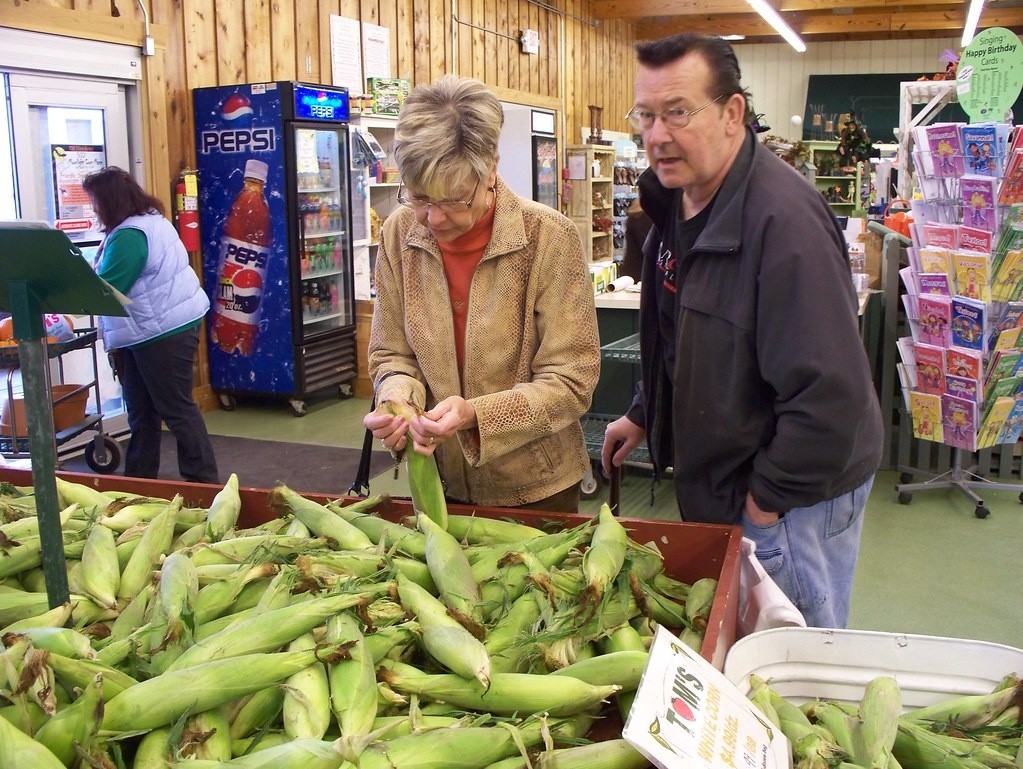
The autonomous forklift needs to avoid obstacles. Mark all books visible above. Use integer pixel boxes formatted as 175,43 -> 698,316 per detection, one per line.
896,122 -> 1023,452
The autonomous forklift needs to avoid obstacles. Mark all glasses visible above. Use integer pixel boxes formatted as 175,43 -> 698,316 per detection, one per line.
397,176 -> 480,211
624,93 -> 727,131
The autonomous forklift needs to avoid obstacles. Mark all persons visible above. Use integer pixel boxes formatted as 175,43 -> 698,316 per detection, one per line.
83,167 -> 220,484
621,198 -> 654,283
603,32 -> 883,629
367,75 -> 602,516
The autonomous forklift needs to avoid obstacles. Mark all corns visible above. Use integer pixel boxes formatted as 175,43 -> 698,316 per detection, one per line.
0,396 -> 718,769
744,672 -> 1023,769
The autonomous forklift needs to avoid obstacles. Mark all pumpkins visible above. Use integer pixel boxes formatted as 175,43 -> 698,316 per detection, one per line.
0,313 -> 74,355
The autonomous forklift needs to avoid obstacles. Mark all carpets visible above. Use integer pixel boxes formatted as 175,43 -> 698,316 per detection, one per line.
53,430 -> 397,494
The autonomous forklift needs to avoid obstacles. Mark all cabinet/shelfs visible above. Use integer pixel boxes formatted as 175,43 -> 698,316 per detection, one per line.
567,144 -> 616,263
892,148 -> 1022,518
348,114 -> 406,299
798,141 -> 861,217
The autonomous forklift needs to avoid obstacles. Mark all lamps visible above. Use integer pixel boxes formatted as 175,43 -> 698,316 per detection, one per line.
520,29 -> 538,54
959,0 -> 986,47
745,0 -> 807,52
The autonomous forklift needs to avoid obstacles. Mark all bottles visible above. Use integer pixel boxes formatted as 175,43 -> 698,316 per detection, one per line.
210,159 -> 271,357
297,154 -> 340,318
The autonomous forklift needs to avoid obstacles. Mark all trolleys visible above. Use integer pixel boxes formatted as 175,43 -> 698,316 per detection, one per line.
0,311 -> 124,475
577,329 -> 673,500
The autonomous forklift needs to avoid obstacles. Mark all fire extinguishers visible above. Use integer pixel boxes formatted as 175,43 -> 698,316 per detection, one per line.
173,165 -> 204,252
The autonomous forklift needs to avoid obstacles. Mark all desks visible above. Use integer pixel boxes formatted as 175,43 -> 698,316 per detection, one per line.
0,466 -> 743,769
594,288 -> 872,480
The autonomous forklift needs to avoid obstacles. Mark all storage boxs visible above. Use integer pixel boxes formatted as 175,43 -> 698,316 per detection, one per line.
367,78 -> 411,115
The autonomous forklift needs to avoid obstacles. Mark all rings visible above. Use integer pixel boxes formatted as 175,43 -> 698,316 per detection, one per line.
427,436 -> 433,446
381,439 -> 392,449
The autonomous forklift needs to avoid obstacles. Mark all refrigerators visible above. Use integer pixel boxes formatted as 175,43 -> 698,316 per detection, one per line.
497,103 -> 559,217
194,81 -> 359,393
